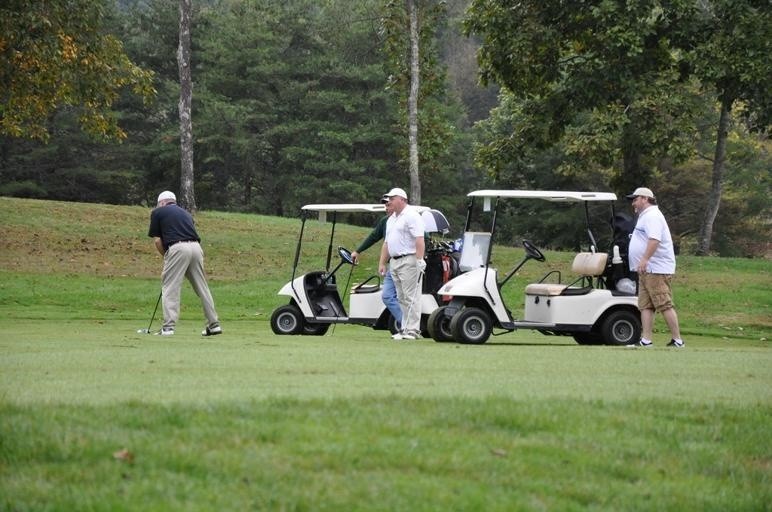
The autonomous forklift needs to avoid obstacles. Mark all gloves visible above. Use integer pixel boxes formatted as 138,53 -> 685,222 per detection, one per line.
417,259 -> 427,274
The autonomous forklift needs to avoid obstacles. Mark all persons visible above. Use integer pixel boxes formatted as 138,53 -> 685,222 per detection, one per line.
147,189 -> 223,337
625,186 -> 686,346
378,187 -> 428,340
350,198 -> 431,324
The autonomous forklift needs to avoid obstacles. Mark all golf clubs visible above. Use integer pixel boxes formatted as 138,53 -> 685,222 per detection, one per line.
144,292 -> 161,333
399,270 -> 423,335
428,227 -> 453,253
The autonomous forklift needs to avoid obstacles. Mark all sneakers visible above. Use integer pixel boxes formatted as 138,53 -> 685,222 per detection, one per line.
155,329 -> 176,336
202,324 -> 222,336
391,330 -> 420,340
626,338 -> 653,347
666,338 -> 685,348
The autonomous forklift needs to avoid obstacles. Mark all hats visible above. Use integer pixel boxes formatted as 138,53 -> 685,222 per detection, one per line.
157,190 -> 177,203
625,187 -> 654,199
380,187 -> 408,203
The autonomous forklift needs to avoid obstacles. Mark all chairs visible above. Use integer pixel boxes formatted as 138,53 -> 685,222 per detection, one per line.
351,275 -> 383,293
524,252 -> 609,297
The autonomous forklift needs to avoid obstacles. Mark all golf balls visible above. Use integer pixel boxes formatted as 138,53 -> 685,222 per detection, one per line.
136,329 -> 141,335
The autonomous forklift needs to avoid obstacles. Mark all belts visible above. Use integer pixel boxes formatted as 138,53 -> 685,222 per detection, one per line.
166,239 -> 183,250
393,254 -> 404,259
